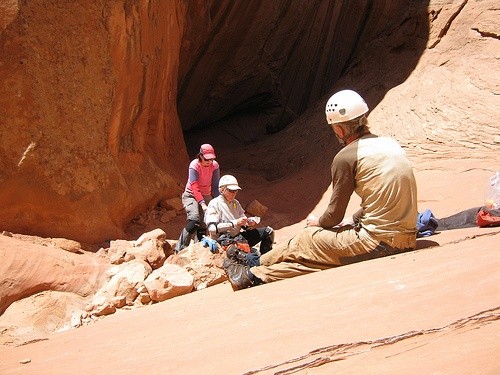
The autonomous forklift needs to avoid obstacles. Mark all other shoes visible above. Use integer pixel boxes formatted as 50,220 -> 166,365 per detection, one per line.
175,250 -> 178,255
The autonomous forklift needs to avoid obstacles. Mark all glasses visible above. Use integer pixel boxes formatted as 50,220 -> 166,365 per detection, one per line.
201,155 -> 214,161
221,187 -> 238,193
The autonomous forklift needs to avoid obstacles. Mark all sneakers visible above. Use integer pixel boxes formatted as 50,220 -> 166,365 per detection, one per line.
226,245 -> 249,266
222,258 -> 254,291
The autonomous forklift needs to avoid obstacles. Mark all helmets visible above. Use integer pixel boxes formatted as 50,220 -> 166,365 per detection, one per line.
325,89 -> 369,125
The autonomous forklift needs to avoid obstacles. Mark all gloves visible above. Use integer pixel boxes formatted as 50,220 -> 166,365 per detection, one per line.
202,237 -> 218,254
265,226 -> 274,234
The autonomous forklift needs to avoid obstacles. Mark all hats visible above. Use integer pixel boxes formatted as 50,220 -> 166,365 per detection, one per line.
200,144 -> 216,159
219,174 -> 242,191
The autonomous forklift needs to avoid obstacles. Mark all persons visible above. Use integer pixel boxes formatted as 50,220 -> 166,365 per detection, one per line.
174,144 -> 220,254
203,175 -> 276,256
223,90 -> 418,292
